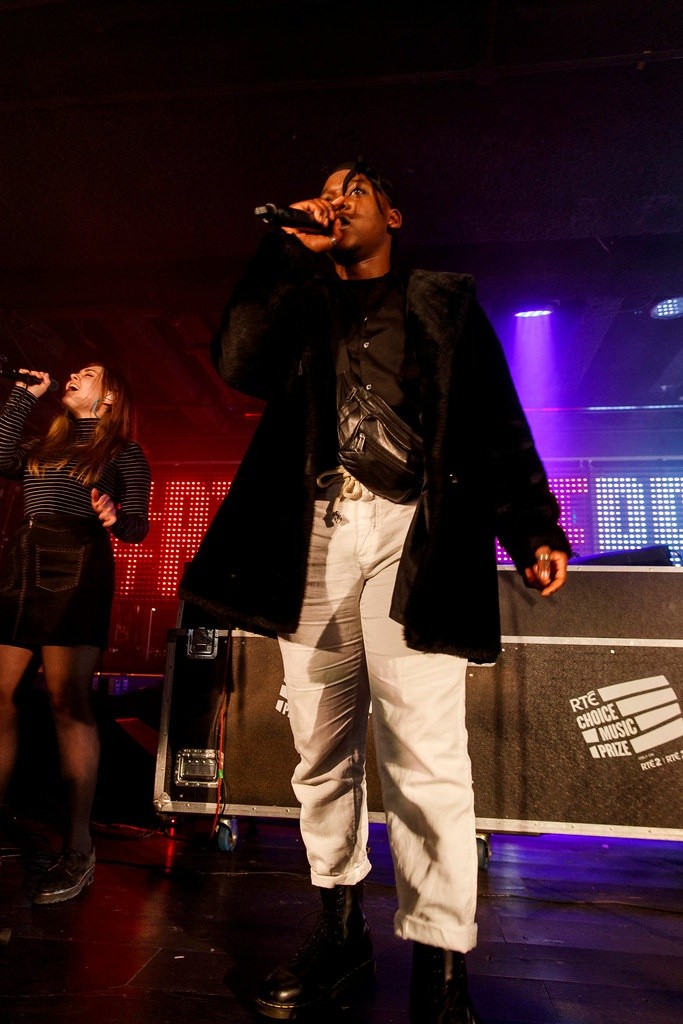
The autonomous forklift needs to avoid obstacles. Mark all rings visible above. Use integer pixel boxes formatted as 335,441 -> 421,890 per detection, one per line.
538,554 -> 550,560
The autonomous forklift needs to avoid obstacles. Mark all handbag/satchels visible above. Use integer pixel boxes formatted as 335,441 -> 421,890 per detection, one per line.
337,386 -> 427,505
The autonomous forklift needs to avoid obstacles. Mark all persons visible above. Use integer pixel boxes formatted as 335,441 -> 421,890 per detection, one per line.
177,162 -> 569,1023
0,360 -> 149,902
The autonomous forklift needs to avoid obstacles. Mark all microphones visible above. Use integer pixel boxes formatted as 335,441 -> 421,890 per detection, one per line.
0,367 -> 59,390
255,201 -> 335,236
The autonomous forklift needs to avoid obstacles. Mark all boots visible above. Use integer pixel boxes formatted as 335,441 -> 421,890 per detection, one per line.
409,943 -> 475,1024
255,880 -> 376,1020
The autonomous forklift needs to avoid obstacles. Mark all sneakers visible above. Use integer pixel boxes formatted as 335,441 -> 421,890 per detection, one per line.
26,842 -> 96,906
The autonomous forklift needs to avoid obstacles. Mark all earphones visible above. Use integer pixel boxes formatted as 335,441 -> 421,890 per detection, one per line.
106,395 -> 114,400
